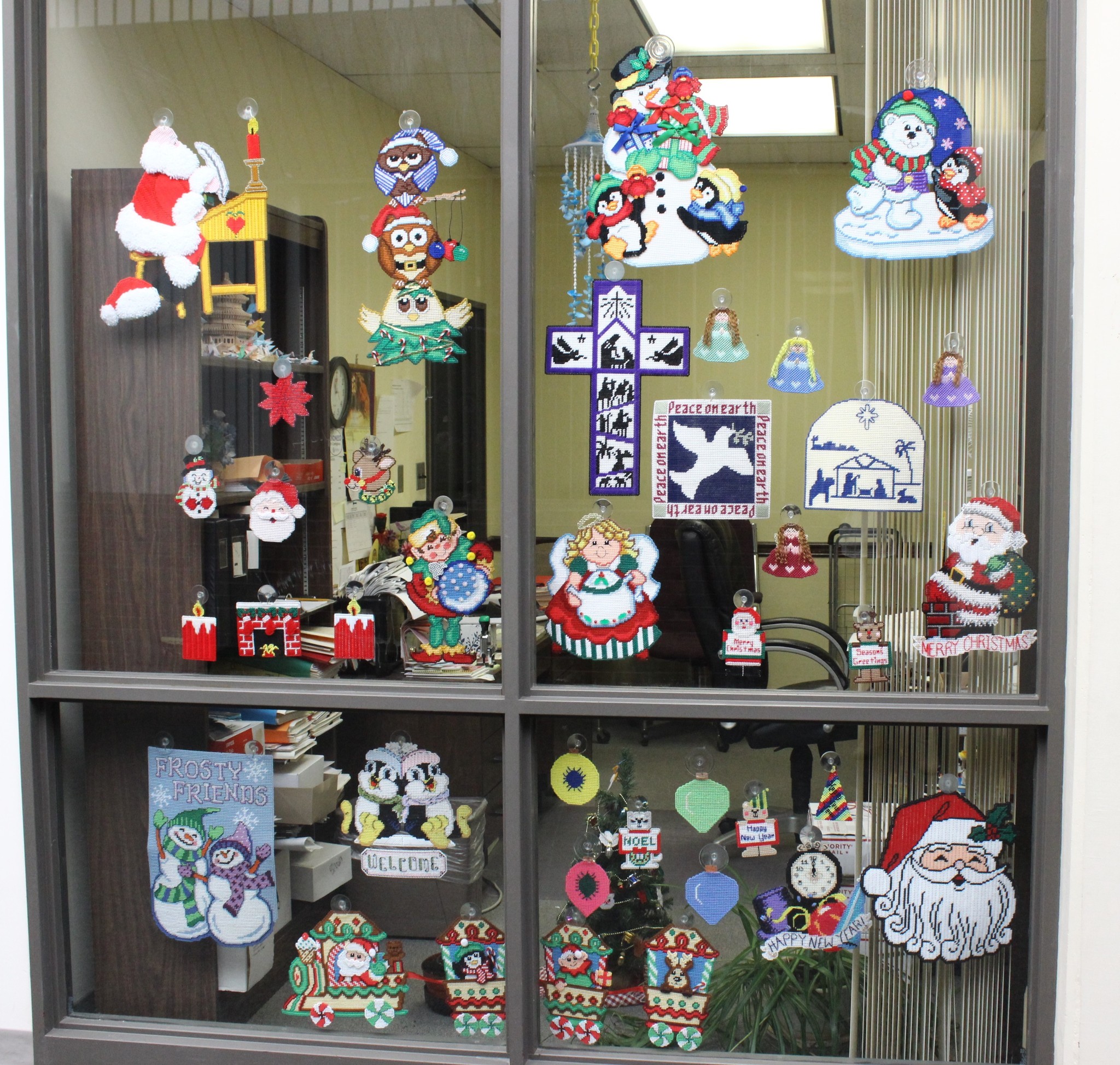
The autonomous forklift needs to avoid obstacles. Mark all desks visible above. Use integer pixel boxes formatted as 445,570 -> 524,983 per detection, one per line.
312,620 -> 592,880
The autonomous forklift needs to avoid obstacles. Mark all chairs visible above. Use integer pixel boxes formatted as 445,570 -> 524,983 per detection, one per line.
390,507 -> 430,524
412,501 -> 434,509
645,520 -> 760,754
674,520 -> 856,847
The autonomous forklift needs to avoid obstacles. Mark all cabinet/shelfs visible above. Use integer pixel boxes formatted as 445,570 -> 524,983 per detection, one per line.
71,166 -> 335,1028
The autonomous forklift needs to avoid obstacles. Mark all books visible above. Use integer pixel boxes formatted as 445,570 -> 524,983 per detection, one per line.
403,664 -> 490,679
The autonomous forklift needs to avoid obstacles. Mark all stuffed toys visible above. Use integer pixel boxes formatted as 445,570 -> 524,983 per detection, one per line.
92,129 -> 232,332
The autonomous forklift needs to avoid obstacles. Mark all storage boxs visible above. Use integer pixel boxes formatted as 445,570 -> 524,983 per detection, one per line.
807,802 -> 900,878
208,719 -> 265,755
274,849 -> 292,934
274,773 -> 338,825
337,592 -> 409,678
217,932 -> 273,993
290,841 -> 352,902
243,597 -> 337,656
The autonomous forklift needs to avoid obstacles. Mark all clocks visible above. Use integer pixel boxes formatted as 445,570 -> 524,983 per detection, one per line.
329,356 -> 352,428
785,841 -> 843,906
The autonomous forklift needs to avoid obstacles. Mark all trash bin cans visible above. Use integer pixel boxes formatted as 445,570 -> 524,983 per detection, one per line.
337,798 -> 488,937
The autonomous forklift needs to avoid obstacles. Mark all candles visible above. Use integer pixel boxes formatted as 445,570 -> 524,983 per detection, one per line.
246,128 -> 261,160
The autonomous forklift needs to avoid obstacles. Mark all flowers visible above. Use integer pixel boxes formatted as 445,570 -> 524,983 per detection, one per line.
374,512 -> 410,542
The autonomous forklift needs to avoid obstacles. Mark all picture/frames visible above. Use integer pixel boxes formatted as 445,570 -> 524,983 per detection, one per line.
342,354 -> 376,501
